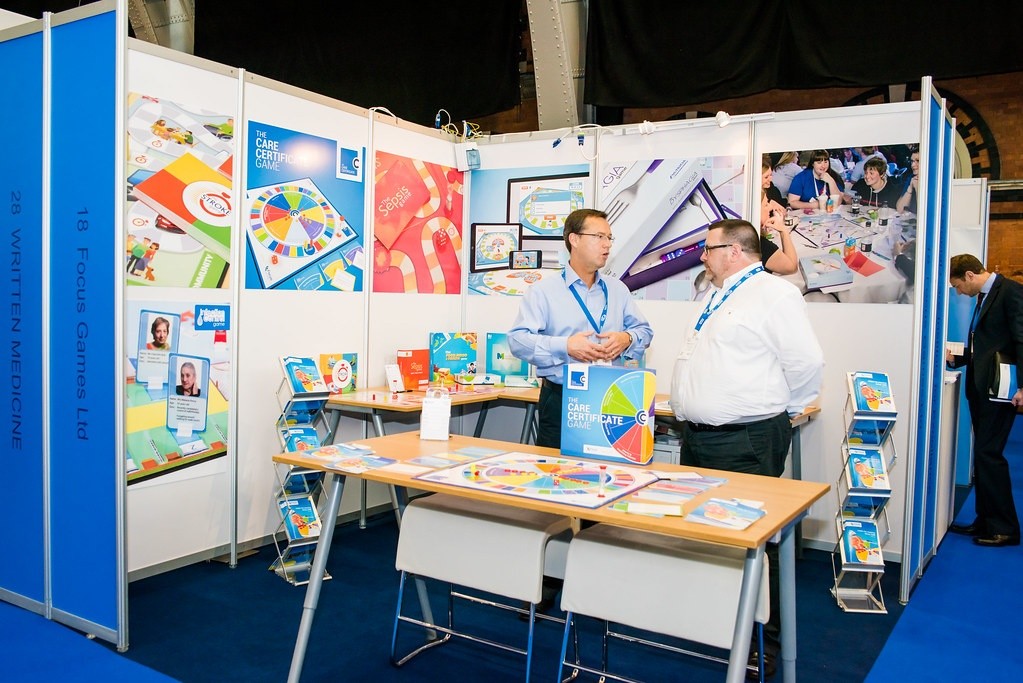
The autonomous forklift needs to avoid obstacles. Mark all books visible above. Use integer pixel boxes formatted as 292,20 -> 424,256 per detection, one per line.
841,518 -> 884,565
610,473 -> 769,532
278,496 -> 321,540
845,447 -> 891,489
849,370 -> 895,413
282,427 -> 322,469
283,355 -> 327,393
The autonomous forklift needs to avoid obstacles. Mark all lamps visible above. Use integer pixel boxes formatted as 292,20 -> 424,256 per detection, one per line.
639,121 -> 654,136
715,111 -> 732,127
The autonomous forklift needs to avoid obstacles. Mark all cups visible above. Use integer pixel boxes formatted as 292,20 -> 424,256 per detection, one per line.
817,196 -> 828,211
830,194 -> 840,211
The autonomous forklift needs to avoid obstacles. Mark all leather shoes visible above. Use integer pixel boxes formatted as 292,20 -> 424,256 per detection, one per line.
747,652 -> 776,679
973,532 -> 1020,546
949,522 -> 983,535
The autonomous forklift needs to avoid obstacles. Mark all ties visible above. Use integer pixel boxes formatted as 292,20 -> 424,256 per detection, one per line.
976,293 -> 986,315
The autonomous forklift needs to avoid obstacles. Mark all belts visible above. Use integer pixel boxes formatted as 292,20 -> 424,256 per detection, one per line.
543,377 -> 562,393
691,423 -> 745,430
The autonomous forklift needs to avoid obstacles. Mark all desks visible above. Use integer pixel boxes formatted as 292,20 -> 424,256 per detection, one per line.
498,388 -> 822,559
273,430 -> 831,683
768,205 -> 917,303
325,386 -> 505,528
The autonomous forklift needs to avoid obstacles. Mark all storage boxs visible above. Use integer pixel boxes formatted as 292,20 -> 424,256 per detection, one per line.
799,253 -> 853,289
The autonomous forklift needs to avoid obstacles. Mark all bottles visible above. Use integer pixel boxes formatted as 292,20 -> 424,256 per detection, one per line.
851,192 -> 861,216
861,222 -> 873,256
877,201 -> 890,238
783,203 -> 794,228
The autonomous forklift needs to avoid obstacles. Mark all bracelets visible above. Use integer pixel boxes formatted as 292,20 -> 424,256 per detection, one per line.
629,336 -> 632,342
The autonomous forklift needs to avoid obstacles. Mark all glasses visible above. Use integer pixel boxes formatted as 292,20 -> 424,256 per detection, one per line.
578,232 -> 616,244
908,159 -> 918,165
704,245 -> 744,256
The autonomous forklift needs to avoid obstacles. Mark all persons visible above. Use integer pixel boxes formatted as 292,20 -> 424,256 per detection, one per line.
671,218 -> 825,683
946,253 -> 1023,547
760,143 -> 919,305
175,361 -> 200,397
147,316 -> 170,351
508,209 -> 654,622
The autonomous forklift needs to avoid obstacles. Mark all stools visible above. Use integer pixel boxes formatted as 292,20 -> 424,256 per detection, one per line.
391,492 -> 582,683
557,523 -> 770,683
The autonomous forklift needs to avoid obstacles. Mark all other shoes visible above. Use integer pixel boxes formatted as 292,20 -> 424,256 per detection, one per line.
520,597 -> 551,623
